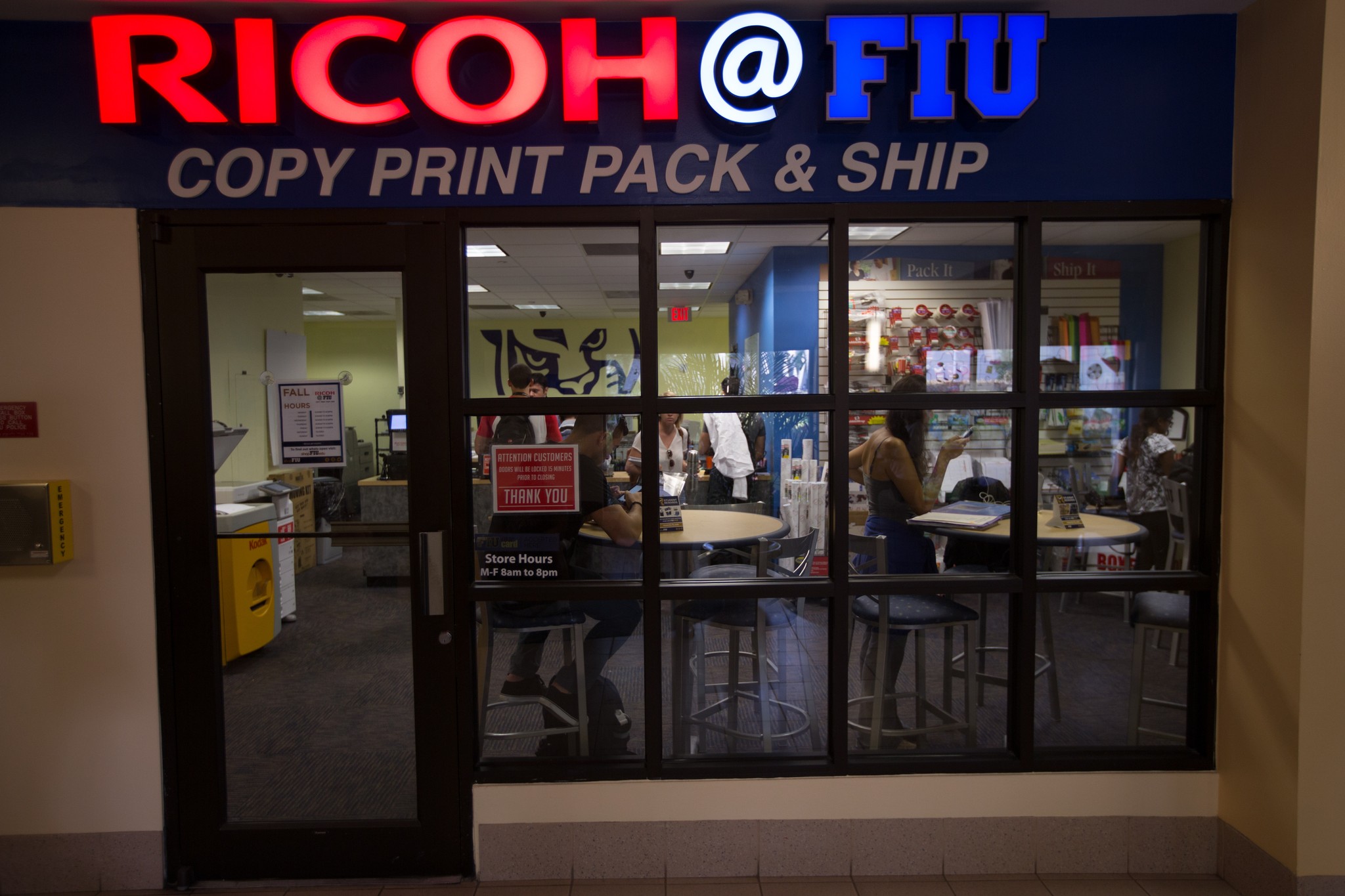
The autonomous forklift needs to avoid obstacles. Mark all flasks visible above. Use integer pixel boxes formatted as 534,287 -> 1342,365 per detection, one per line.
685,450 -> 698,493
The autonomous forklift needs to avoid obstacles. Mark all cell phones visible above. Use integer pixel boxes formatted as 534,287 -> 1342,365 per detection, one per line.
961,428 -> 973,438
617,483 -> 642,504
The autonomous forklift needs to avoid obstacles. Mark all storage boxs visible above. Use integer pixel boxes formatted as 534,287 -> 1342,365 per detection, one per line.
267,467 -> 317,574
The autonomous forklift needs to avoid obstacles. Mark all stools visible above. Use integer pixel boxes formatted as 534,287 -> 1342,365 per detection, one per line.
673,526 -> 820,753
845,531 -> 1060,755
1131,592 -> 1190,748
477,610 -> 589,759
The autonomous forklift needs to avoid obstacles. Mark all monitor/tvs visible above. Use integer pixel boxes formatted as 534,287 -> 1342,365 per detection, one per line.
389,414 -> 406,430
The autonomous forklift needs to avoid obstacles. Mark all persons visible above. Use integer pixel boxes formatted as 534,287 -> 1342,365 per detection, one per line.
474,362 -> 575,454
625,413 -> 690,503
1108,407 -> 1186,570
698,376 -> 767,566
482,414 -> 644,707
848,375 -> 972,752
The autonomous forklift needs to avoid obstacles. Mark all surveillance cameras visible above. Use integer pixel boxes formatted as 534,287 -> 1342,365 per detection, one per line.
684,270 -> 694,279
540,311 -> 546,318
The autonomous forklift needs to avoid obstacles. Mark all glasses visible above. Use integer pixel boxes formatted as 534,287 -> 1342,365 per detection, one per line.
667,450 -> 675,468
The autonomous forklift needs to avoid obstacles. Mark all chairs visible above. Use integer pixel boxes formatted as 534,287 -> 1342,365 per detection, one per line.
1161,478 -> 1191,595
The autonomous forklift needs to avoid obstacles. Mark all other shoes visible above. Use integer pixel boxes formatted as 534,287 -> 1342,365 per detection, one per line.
858,740 -> 916,753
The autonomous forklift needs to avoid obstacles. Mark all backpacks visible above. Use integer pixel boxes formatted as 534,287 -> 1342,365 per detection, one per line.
493,415 -> 536,445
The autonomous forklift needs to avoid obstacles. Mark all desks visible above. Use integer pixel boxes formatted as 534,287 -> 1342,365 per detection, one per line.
579,509 -> 790,760
922,509 -> 1148,754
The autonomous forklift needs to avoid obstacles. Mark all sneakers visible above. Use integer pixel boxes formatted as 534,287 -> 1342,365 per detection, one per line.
499,673 -> 547,702
538,685 -> 579,726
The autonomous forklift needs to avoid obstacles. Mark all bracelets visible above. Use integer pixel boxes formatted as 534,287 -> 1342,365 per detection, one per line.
630,502 -> 642,509
